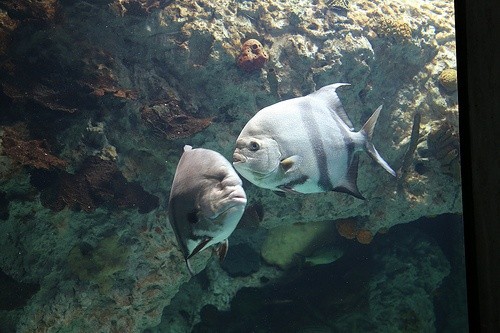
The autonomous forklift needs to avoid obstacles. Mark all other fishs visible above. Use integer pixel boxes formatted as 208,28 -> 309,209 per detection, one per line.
167,144 -> 248,264
233,82 -> 397,202
304,247 -> 345,265
